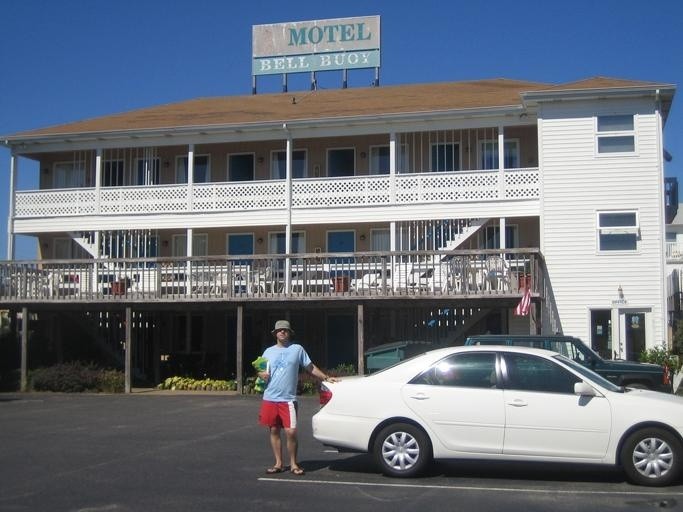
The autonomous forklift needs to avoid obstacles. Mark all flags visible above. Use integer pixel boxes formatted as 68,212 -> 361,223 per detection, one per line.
514,290 -> 531,315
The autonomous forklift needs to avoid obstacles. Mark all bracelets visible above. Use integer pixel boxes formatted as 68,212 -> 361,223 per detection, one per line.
325,376 -> 329,382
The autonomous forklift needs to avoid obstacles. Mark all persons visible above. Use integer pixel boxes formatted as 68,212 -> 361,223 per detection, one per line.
256,320 -> 342,475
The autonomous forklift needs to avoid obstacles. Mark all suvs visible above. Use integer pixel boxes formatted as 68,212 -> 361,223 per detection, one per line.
463,333 -> 673,395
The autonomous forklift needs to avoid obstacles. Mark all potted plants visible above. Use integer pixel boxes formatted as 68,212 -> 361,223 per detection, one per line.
517,260 -> 534,291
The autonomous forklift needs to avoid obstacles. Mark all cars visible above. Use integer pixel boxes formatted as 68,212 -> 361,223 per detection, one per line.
311,344 -> 682,488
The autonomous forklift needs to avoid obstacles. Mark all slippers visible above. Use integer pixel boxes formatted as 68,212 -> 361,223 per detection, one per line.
266,466 -> 284,473
290,468 -> 304,475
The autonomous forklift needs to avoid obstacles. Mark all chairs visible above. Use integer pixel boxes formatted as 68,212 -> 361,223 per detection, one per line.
189,266 -> 275,297
351,271 -> 430,295
441,254 -> 511,293
0,270 -> 63,299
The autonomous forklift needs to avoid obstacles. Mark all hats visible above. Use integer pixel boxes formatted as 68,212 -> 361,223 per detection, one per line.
270,320 -> 295,337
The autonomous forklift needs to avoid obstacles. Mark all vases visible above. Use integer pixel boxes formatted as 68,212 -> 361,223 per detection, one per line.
332,276 -> 351,292
111,281 -> 126,295
